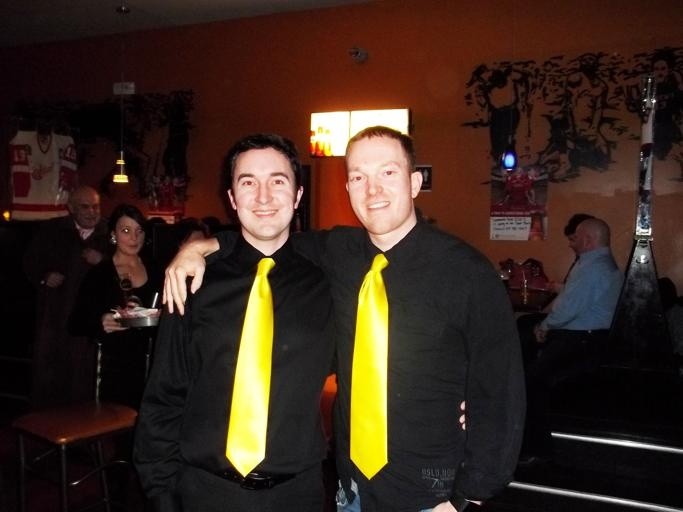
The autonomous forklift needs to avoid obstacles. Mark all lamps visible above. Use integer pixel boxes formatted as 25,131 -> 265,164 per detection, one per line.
112,4 -> 130,184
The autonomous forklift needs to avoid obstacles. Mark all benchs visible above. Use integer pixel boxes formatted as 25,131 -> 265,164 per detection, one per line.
538,235 -> 682,447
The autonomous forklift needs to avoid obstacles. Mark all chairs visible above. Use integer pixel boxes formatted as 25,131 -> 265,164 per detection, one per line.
9,337 -> 154,512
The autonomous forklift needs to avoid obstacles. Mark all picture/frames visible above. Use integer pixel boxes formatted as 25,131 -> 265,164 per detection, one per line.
415,165 -> 432,192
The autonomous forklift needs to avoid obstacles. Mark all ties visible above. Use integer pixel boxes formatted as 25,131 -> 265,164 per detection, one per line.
350,253 -> 391,483
224,257 -> 276,482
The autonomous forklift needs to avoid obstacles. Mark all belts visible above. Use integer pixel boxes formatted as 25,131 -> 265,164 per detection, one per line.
182,455 -> 312,493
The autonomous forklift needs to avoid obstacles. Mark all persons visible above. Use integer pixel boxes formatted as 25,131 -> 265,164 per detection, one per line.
516,213 -> 597,356
67,204 -> 165,510
129,131 -> 468,512
22,185 -> 111,412
516,218 -> 624,467
158,126 -> 528,512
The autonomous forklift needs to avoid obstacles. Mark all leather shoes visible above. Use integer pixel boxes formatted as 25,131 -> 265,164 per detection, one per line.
518,430 -> 569,466
